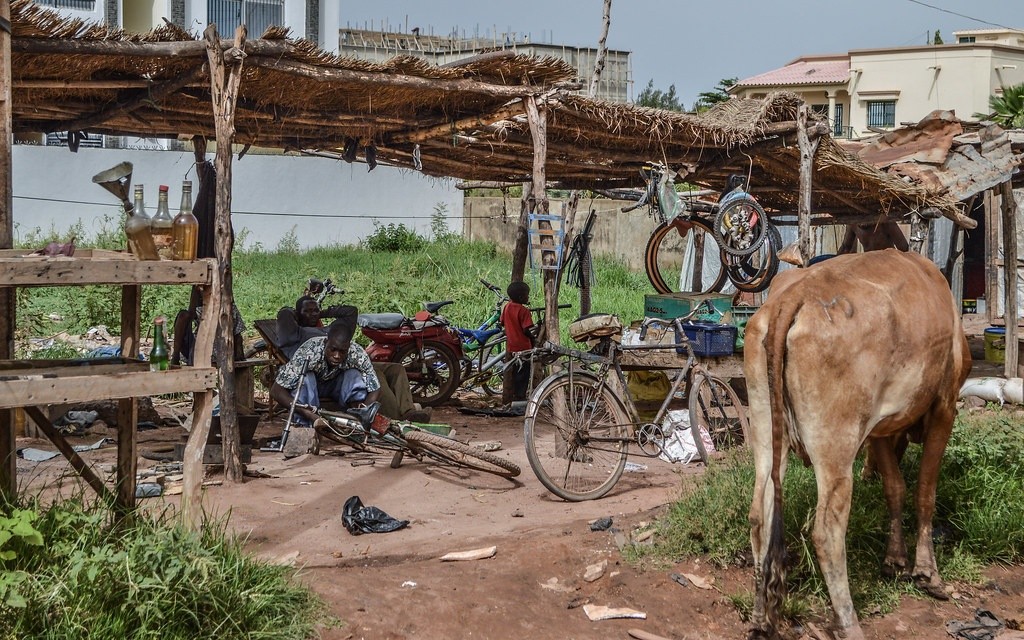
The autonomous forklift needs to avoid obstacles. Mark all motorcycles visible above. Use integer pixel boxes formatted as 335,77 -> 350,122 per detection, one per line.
242,276 -> 466,409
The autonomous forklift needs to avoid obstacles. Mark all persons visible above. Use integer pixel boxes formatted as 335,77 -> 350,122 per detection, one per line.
270,323 -> 380,429
837,219 -> 910,257
500,281 -> 537,403
303,288 -> 309,297
275,296 -> 430,423
169,284 -> 246,368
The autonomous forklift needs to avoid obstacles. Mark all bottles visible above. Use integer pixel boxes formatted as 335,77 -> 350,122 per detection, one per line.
150,318 -> 168,372
161,319 -> 171,370
171,181 -> 199,261
133,183 -> 152,236
152,185 -> 175,262
123,200 -> 159,260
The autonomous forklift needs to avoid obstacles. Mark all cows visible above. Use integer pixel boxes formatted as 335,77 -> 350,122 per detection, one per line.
742,246 -> 974,640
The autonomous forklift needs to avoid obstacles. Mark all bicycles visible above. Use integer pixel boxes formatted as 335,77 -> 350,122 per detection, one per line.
414,277 -> 522,399
521,300 -> 752,500
279,354 -> 521,481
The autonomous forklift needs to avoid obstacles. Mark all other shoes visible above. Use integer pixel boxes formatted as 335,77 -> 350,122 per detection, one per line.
399,406 -> 432,424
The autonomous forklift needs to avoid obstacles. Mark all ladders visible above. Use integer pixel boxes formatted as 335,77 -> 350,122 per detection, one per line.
527,200 -> 567,298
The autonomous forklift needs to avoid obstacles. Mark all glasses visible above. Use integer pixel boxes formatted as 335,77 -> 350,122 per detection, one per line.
303,308 -> 321,314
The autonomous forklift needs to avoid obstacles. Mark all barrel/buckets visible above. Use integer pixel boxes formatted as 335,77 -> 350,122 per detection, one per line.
984,327 -> 1007,367
